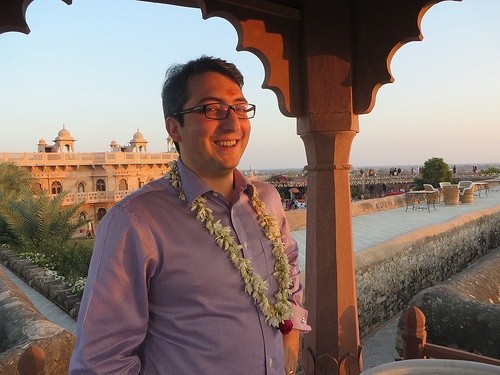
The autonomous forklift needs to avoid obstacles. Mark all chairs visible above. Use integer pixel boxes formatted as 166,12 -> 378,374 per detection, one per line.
439,182 -> 452,202
423,184 -> 440,204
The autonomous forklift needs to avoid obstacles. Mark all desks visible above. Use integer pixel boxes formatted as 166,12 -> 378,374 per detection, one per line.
404,190 -> 437,213
485,180 -> 500,193
438,186 -> 465,204
473,183 -> 489,199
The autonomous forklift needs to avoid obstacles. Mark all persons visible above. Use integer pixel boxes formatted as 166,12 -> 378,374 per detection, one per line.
67,56 -> 307,374
268,161 -> 481,211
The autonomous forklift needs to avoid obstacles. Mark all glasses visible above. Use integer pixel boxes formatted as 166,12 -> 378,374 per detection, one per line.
171,103 -> 256,119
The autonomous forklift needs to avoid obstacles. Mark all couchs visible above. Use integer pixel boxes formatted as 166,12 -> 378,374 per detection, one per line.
442,186 -> 460,205
457,181 -> 474,203
